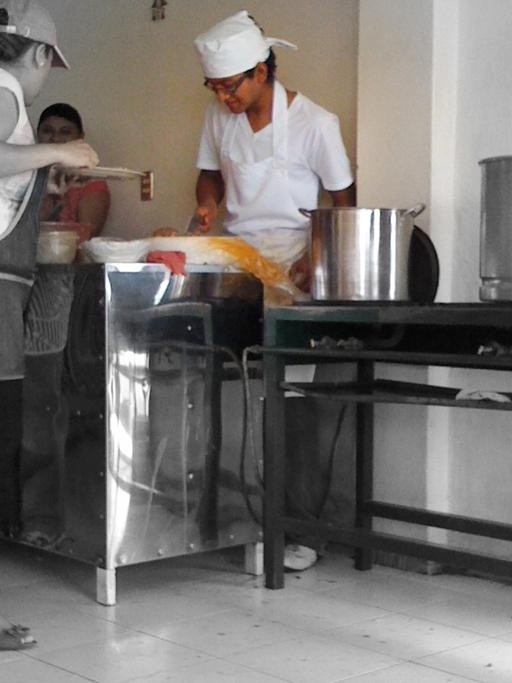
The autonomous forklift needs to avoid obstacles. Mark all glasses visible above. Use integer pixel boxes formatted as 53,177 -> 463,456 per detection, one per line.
204,75 -> 248,94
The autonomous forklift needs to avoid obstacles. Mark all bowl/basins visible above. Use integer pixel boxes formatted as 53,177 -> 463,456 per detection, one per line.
104,266 -> 266,361
32,220 -> 82,264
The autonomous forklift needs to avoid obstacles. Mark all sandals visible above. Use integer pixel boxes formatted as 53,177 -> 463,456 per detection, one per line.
0,624 -> 36,651
20,529 -> 64,549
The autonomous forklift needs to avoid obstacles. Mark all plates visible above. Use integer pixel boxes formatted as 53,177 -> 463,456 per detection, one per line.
51,163 -> 147,182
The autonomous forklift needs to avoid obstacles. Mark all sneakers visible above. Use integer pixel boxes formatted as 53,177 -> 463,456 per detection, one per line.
284,543 -> 319,571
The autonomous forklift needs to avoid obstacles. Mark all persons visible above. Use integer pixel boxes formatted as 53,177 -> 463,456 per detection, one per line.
180,8 -> 356,575
0,0 -> 102,652
33,99 -> 114,242
15,265 -> 82,550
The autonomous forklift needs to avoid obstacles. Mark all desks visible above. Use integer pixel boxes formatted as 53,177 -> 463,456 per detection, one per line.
261,297 -> 511,591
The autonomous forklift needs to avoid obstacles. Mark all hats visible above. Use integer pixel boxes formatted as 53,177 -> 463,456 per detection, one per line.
193,9 -> 270,80
0,0 -> 71,72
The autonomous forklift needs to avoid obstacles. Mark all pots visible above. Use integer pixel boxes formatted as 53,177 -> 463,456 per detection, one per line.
478,153 -> 512,304
294,202 -> 429,304
